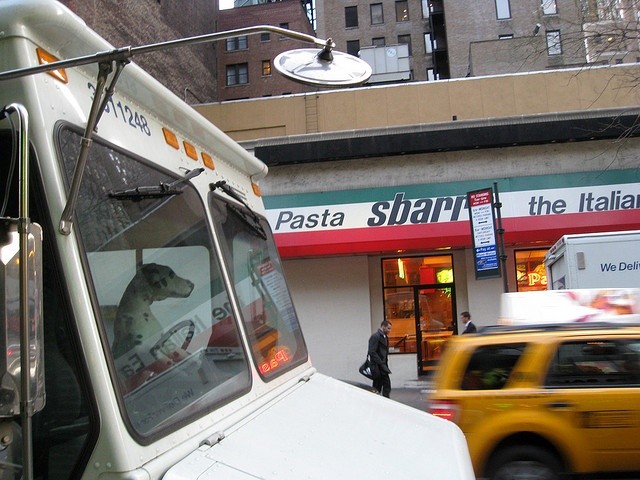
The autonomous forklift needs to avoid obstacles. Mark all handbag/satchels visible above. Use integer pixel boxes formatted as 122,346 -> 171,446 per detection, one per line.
359,360 -> 373,379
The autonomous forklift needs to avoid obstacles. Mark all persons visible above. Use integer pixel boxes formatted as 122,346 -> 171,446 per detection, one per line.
461,312 -> 476,336
355,320 -> 393,398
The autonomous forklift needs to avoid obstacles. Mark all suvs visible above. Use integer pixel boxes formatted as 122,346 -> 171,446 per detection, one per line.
422,322 -> 640,478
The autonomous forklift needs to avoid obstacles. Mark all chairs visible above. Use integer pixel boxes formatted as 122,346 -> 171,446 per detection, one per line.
29,194 -> 91,478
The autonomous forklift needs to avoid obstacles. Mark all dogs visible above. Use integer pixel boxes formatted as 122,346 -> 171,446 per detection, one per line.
108,262 -> 204,395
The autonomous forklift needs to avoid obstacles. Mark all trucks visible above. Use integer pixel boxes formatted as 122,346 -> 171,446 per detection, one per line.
1,1 -> 480,478
541,229 -> 639,288
